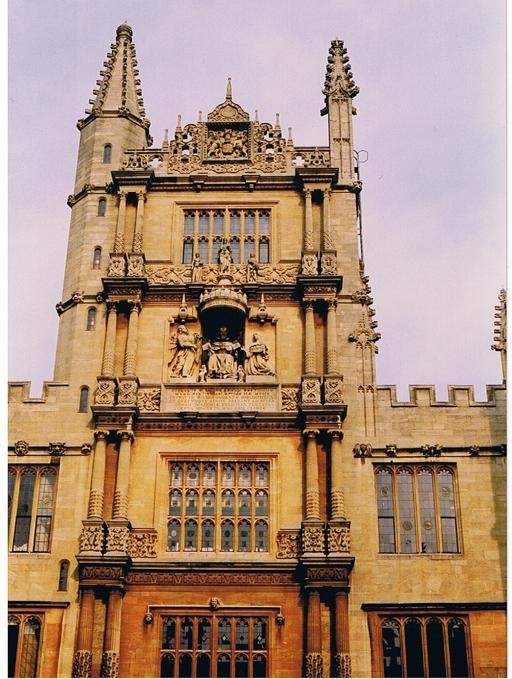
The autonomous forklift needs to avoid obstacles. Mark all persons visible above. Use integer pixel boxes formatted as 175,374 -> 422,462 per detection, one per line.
248,332 -> 274,376
168,325 -> 201,378
204,323 -> 241,380
